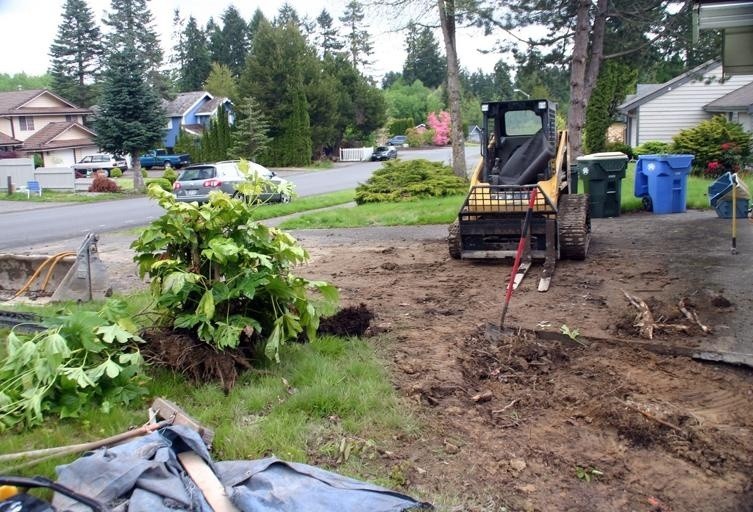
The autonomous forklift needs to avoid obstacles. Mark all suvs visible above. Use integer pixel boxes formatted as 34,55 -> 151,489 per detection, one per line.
68,152 -> 128,178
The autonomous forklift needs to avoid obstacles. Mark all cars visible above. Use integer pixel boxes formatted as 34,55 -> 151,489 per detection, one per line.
386,135 -> 407,146
370,145 -> 397,162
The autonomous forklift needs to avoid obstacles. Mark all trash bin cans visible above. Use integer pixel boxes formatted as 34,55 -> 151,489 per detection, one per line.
633,154 -> 694,215
577,149 -> 629,219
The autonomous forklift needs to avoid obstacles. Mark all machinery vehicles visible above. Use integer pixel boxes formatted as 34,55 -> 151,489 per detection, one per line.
444,97 -> 592,296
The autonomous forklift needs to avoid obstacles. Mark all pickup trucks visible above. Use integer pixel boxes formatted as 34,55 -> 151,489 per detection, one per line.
131,146 -> 190,171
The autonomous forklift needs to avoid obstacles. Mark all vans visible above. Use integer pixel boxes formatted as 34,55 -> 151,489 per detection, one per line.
171,158 -> 292,207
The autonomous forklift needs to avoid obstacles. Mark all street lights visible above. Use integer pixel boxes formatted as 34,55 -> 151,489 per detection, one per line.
511,88 -> 529,99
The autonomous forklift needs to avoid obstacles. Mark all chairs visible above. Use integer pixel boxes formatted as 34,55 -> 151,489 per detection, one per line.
26,181 -> 41,200
